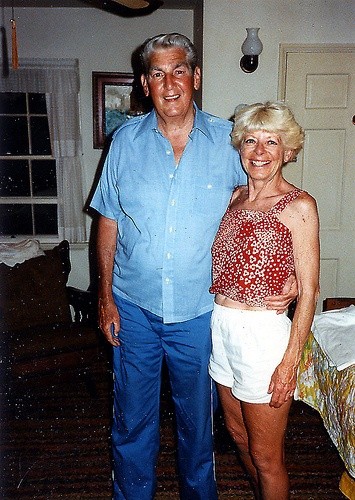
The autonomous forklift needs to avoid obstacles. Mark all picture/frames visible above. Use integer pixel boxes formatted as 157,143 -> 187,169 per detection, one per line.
92,71 -> 147,149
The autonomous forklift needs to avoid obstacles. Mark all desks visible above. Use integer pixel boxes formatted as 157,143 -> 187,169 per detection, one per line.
294,304 -> 355,500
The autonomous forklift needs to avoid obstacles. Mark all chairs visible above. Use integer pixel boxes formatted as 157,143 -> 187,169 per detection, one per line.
0,240 -> 97,399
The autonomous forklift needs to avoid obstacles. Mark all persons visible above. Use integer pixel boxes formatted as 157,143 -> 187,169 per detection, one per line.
208,102 -> 319,500
89,33 -> 300,500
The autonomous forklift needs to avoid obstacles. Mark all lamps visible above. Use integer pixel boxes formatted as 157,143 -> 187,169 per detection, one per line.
240,27 -> 263,73
